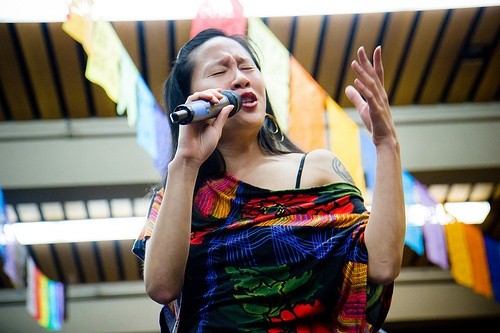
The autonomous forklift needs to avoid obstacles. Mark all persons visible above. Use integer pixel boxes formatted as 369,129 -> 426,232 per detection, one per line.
131,29 -> 406,333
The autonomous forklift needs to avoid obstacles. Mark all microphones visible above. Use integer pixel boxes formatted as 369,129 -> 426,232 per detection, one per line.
170,90 -> 242,125
